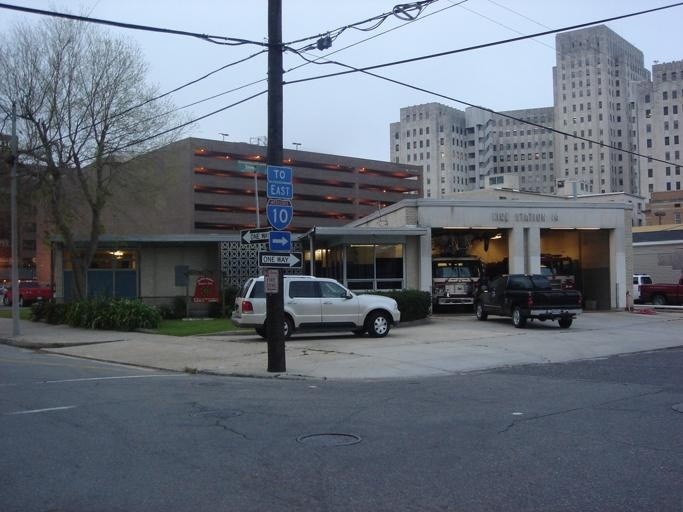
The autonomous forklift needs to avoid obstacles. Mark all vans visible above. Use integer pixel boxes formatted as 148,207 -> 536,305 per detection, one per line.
633,274 -> 653,303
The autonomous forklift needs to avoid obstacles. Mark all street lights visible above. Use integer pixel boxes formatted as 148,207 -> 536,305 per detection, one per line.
654,211 -> 664,225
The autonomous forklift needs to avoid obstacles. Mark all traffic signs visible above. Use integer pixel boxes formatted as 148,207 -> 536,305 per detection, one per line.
240,227 -> 274,244
257,251 -> 302,269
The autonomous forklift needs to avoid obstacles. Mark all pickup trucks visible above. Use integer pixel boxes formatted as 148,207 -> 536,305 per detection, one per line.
3,281 -> 51,308
637,276 -> 682,307
475,273 -> 582,330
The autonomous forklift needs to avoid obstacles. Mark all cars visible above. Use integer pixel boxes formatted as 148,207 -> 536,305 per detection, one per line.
0,285 -> 8,295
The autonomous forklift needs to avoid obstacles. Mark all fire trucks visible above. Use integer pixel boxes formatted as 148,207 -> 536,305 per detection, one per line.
498,253 -> 576,290
430,253 -> 485,315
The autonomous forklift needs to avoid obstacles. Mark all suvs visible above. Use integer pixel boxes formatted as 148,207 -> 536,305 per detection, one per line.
232,272 -> 401,341
